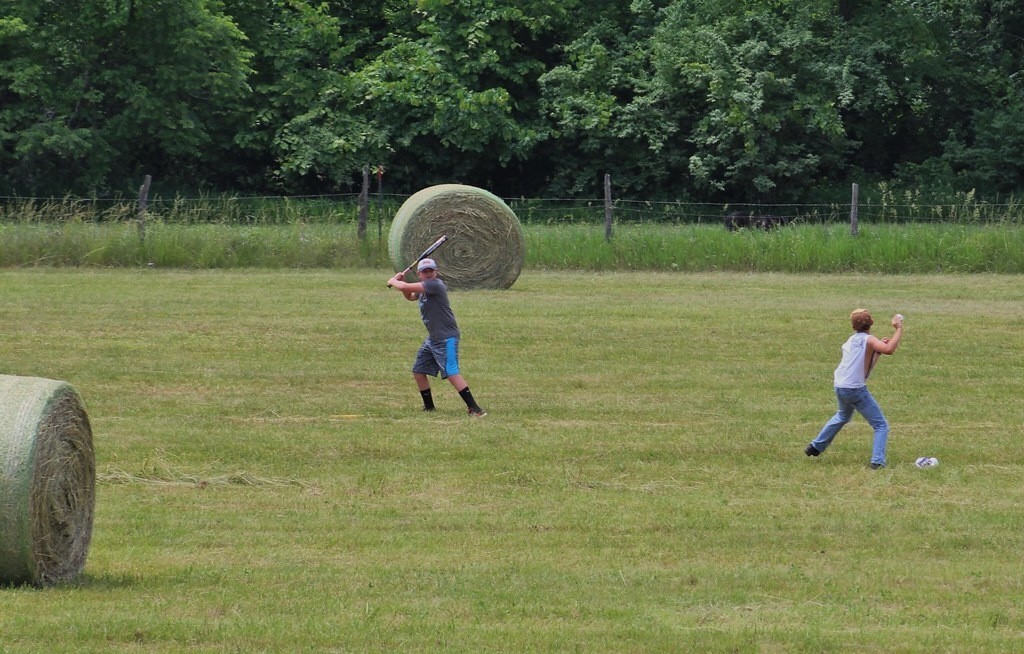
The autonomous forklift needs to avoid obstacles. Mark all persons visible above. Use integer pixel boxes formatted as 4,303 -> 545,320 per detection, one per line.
388,259 -> 486,418
804,309 -> 902,469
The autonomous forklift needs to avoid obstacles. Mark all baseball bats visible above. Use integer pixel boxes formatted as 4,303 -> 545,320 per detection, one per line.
386,235 -> 450,289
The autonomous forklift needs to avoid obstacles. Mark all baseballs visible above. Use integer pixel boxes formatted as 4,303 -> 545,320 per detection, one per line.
896,313 -> 904,322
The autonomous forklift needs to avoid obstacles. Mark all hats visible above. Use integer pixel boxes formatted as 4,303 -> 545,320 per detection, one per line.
417,258 -> 439,272
851,308 -> 874,331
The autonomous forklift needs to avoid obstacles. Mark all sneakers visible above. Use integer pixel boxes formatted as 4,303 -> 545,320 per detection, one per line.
805,444 -> 820,458
871,463 -> 884,469
466,408 -> 488,419
422,405 -> 436,411
